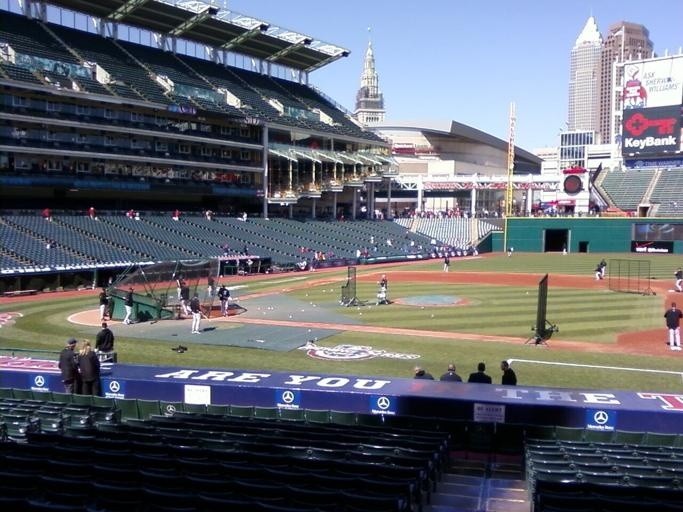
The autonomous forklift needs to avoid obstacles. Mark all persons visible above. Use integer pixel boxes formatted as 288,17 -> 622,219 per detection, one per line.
41,208 -> 51,220
664,302 -> 683,348
126,209 -> 136,219
407,204 -> 599,218
242,211 -> 249,220
58,323 -> 115,396
377,274 -> 389,305
223,243 -> 230,256
506,246 -> 513,257
673,266 -> 683,292
298,232 -> 451,260
121,287 -> 135,326
88,206 -> 96,219
562,241 -> 568,256
207,270 -> 229,318
440,364 -> 463,381
99,287 -> 109,322
468,363 -> 492,384
444,255 -> 450,272
176,272 -> 201,334
173,209 -> 181,221
242,244 -> 249,256
414,367 -> 434,379
501,361 -> 517,385
204,210 -> 213,220
594,258 -> 606,281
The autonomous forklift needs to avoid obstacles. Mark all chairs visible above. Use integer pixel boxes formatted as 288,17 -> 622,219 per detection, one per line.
522,437 -> 683,512
